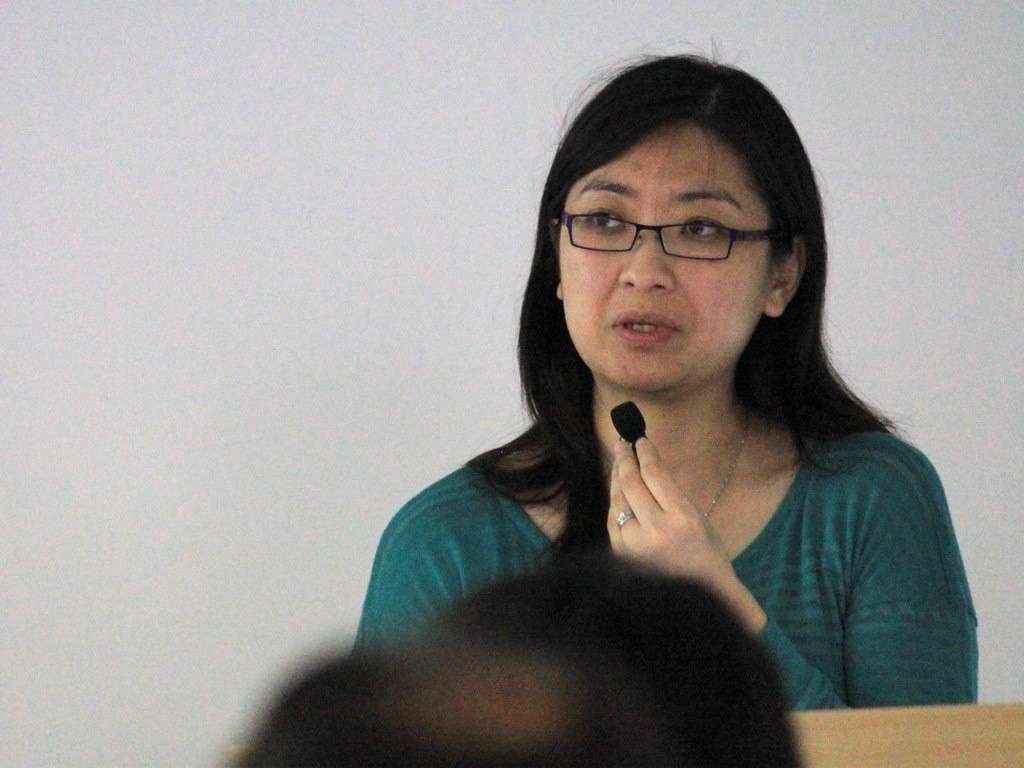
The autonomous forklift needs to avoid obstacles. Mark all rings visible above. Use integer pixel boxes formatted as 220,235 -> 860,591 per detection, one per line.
618,508 -> 637,532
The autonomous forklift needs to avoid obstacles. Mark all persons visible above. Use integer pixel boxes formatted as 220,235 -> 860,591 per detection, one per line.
346,53 -> 980,712
219,635 -> 681,768
427,558 -> 803,768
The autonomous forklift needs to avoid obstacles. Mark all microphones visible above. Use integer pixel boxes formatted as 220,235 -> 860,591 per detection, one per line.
611,401 -> 646,451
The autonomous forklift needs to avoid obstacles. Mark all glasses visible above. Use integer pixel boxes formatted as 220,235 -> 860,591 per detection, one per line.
561,211 -> 772,261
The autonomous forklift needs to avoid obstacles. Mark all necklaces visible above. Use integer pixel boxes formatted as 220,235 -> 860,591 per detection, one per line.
695,399 -> 763,532
